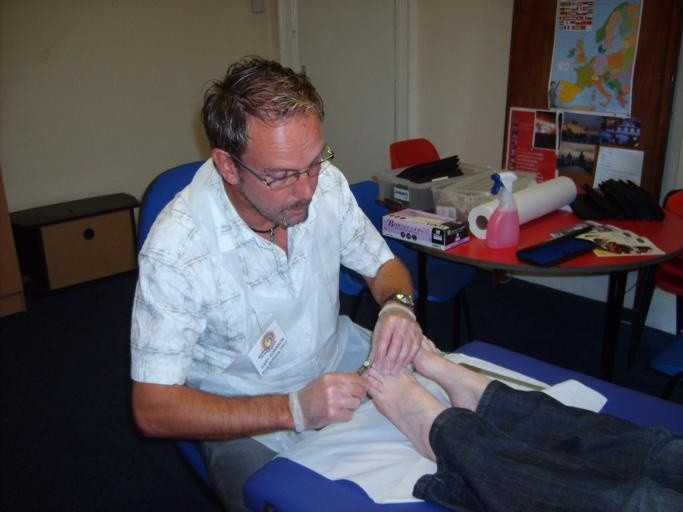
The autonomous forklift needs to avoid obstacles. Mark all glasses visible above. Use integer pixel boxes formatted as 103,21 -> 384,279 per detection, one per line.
230,143 -> 335,191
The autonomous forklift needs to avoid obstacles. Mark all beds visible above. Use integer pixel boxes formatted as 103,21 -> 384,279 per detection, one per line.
242,341 -> 682,511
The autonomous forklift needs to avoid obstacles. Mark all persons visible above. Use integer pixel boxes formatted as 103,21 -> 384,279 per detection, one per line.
130,57 -> 423,511
365,335 -> 682,512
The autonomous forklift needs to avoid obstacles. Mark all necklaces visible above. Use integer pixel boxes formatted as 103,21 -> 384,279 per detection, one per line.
249,223 -> 279,241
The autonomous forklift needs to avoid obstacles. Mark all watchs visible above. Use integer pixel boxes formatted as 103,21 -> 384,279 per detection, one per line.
384,292 -> 417,308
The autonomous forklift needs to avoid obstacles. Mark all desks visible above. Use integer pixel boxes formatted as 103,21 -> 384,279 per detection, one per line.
390,188 -> 683,381
9,193 -> 140,291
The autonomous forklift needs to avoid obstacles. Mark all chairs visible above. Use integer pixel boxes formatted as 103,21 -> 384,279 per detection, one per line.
337,182 -> 478,353
627,187 -> 683,370
390,139 -> 440,170
134,160 -> 230,488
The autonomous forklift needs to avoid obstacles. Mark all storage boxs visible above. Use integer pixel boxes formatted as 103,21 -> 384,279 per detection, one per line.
431,167 -> 536,222
376,162 -> 496,211
